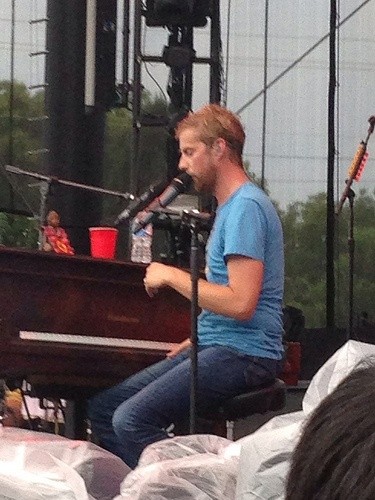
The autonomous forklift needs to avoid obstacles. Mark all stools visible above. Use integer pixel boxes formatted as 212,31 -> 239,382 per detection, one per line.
200,378 -> 288,441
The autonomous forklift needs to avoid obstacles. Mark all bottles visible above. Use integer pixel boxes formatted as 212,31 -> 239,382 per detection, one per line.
131,201 -> 153,264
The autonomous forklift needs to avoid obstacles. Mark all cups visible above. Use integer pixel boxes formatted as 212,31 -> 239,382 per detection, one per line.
89,227 -> 119,260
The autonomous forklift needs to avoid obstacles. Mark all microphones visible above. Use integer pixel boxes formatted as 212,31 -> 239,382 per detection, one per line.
131,173 -> 192,234
112,174 -> 172,227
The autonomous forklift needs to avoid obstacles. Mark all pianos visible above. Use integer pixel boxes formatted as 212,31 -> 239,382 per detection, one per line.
1,246 -> 302,438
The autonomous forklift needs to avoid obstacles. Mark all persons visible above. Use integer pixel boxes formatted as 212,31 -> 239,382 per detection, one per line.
88,106 -> 284,469
42,211 -> 76,256
